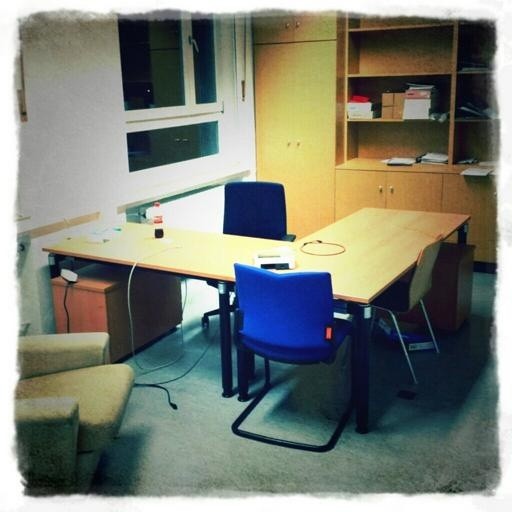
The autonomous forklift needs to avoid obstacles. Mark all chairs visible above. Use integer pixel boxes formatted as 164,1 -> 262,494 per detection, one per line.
232,262 -> 357,453
371,237 -> 444,385
13,332 -> 134,497
202,182 -> 296,327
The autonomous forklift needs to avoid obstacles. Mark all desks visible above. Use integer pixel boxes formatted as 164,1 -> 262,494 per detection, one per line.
43,208 -> 471,398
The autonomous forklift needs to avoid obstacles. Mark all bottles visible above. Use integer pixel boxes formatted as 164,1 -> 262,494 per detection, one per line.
152,202 -> 164,238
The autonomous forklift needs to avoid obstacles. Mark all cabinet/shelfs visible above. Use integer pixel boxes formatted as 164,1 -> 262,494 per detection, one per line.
51,261 -> 182,364
390,243 -> 476,336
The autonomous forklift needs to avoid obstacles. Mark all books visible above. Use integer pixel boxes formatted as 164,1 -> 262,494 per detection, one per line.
454,136 -> 497,178
386,152 -> 448,168
